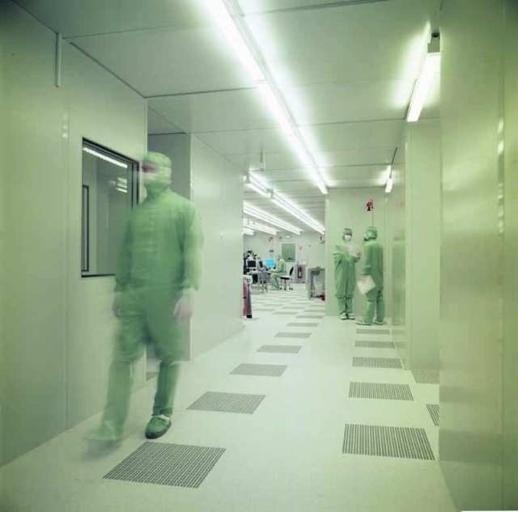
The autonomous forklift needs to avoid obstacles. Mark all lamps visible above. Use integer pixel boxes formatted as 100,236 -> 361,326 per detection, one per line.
402,30 -> 440,124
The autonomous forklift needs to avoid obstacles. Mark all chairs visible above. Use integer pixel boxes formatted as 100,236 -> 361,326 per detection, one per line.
251,272 -> 268,293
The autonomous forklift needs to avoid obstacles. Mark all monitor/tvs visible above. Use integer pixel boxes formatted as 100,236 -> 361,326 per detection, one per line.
246,260 -> 257,272
258,260 -> 264,270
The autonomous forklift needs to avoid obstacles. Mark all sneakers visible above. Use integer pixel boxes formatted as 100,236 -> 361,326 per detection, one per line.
143,415 -> 172,438
357,319 -> 384,325
340,312 -> 356,319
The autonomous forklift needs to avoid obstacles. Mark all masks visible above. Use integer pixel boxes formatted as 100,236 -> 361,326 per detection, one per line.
344,234 -> 352,240
140,171 -> 159,183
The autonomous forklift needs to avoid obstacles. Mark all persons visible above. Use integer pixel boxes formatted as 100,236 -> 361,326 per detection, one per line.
357,227 -> 386,326
268,255 -> 286,289
83,149 -> 202,444
332,227 -> 361,321
